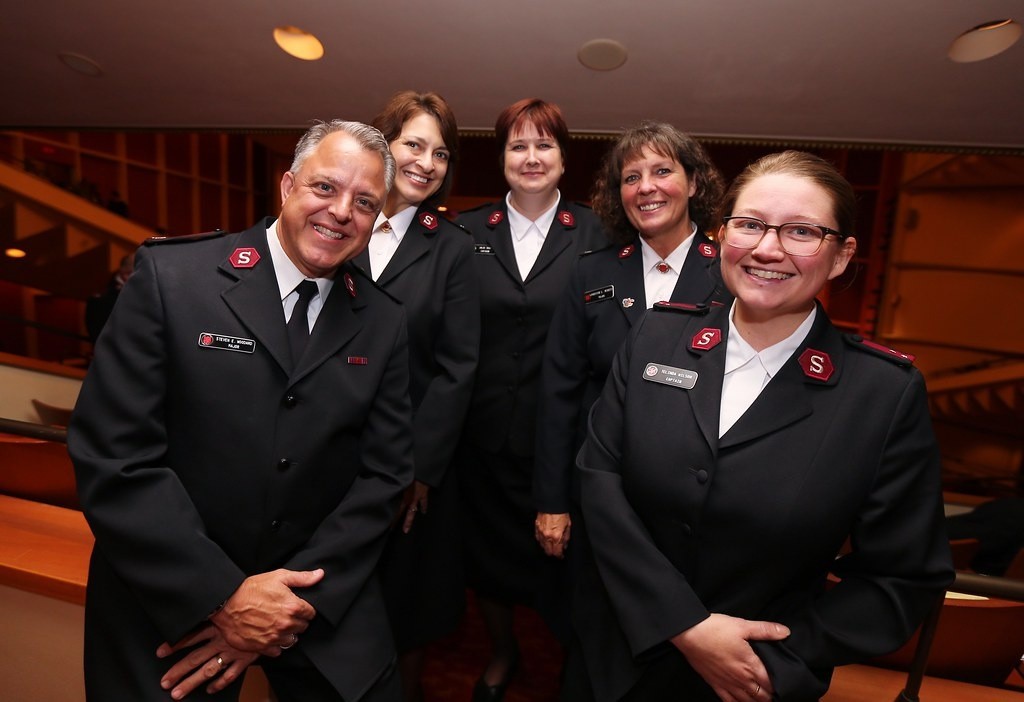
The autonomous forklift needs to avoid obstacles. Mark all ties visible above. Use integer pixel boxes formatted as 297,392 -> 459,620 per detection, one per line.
284,279 -> 320,365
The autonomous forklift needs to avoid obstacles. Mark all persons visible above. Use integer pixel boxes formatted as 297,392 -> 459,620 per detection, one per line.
576,152 -> 957,702
67,90 -> 483,702
458,99 -> 723,702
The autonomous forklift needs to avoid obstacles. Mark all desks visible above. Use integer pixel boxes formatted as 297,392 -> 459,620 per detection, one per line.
0,493 -> 97,702
819,663 -> 1024,702
826,566 -> 1024,687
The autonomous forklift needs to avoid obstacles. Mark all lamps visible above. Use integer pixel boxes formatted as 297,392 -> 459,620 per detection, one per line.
946,19 -> 1022,65
273,24 -> 324,62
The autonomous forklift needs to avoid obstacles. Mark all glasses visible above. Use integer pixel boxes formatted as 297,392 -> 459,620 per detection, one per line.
721,214 -> 842,256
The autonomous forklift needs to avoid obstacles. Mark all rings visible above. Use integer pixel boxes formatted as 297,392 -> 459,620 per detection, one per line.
753,685 -> 760,697
216,656 -> 227,669
281,635 -> 298,649
